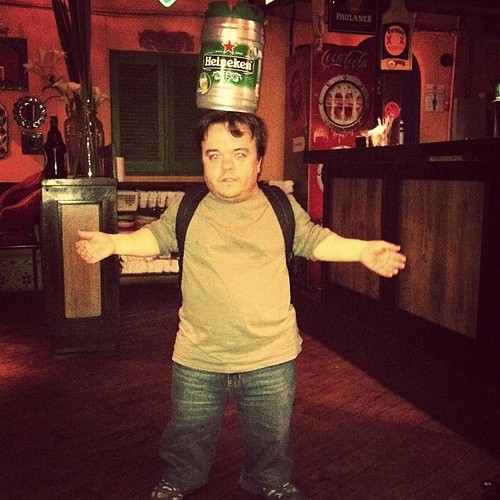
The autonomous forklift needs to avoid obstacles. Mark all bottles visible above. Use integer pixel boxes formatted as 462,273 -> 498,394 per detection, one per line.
40,116 -> 67,180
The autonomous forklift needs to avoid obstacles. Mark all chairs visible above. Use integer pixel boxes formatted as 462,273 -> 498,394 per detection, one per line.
0,170 -> 43,293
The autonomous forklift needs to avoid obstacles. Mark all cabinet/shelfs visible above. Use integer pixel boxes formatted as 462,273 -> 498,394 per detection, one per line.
40,143 -> 121,340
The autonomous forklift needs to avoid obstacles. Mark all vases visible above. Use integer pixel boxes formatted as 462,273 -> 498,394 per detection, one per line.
60,113 -> 105,176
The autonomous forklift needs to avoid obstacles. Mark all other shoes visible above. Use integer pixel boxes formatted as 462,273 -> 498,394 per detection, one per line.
240,475 -> 306,500
150,474 -> 186,499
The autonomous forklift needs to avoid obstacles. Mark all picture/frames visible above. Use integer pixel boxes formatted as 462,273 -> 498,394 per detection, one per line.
0,104 -> 8,158
0,37 -> 28,91
12,96 -> 46,128
22,132 -> 44,154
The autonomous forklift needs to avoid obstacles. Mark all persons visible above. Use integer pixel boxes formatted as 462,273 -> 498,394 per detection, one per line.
75,110 -> 406,500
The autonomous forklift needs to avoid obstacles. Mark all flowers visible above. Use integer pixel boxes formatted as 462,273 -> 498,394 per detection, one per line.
23,44 -> 110,112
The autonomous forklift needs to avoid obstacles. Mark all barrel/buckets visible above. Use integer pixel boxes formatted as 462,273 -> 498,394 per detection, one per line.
194,1 -> 265,114
